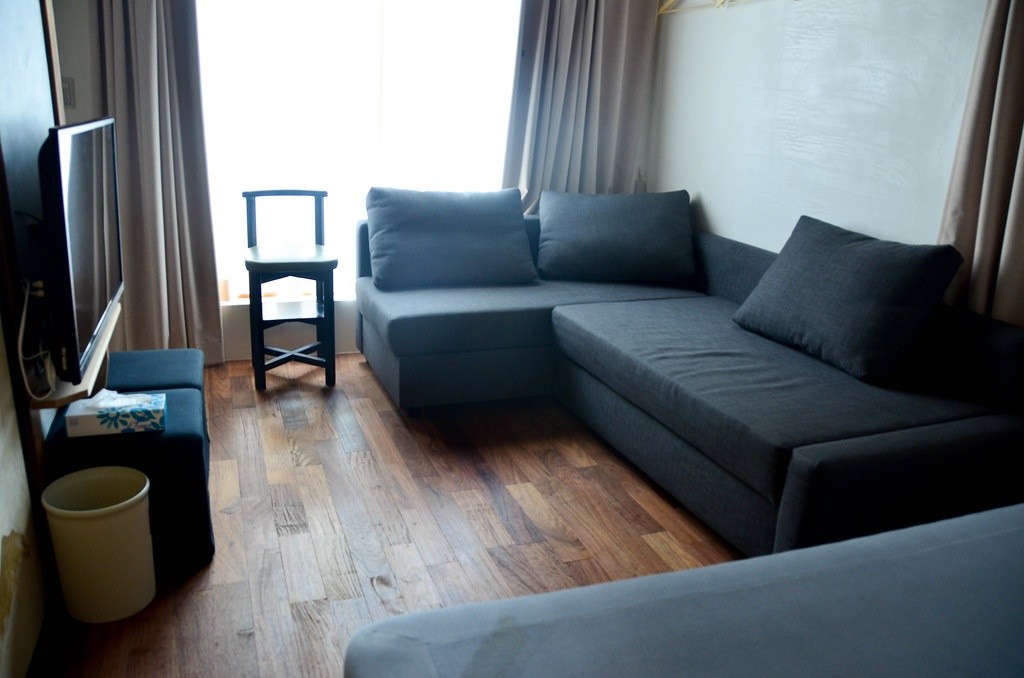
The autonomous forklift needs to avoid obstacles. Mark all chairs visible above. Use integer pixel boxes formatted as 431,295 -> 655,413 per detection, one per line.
241,188 -> 339,392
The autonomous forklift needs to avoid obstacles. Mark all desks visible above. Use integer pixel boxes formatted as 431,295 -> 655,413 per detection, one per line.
344,503 -> 1024,678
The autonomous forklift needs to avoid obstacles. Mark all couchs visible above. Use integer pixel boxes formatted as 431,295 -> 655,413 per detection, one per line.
353,215 -> 1024,558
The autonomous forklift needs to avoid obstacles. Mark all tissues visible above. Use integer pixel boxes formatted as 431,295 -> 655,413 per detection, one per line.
64,387 -> 169,440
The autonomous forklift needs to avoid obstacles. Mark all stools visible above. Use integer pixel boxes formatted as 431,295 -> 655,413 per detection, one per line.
42,387 -> 216,574
107,347 -> 211,446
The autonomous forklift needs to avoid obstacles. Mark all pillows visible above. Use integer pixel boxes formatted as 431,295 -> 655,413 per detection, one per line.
537,189 -> 698,288
732,215 -> 965,386
366,186 -> 539,290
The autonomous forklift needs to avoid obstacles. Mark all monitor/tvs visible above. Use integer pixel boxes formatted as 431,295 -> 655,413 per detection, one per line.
39,116 -> 126,386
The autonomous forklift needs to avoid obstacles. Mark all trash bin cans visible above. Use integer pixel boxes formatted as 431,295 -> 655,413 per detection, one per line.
43,466 -> 156,625
